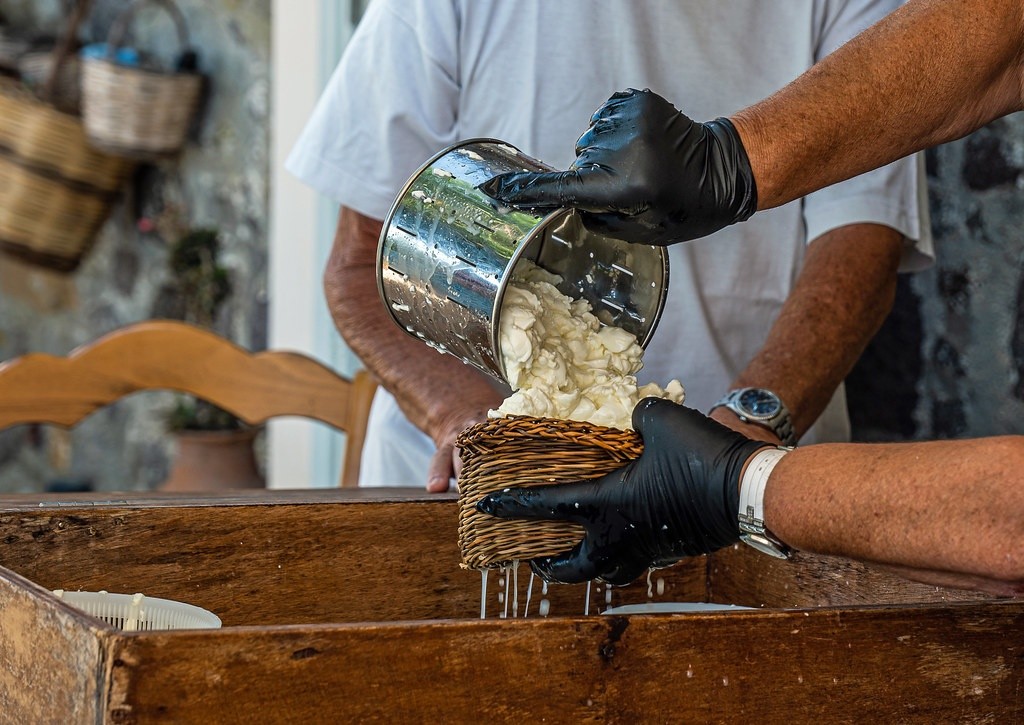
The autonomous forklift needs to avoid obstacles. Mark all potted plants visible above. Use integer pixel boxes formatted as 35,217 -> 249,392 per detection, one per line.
144,226 -> 265,496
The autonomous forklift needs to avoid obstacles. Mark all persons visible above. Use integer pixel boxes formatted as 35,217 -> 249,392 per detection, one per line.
475,0 -> 1024,587
284,0 -> 937,492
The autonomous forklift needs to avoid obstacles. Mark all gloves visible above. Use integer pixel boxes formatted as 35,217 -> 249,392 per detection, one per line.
478,88 -> 758,247
476,397 -> 779,582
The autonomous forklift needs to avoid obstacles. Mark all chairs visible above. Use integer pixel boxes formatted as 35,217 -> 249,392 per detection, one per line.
0,316 -> 381,491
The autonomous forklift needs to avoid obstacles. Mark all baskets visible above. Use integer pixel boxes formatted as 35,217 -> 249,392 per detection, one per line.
453,414 -> 645,571
0,1 -> 203,276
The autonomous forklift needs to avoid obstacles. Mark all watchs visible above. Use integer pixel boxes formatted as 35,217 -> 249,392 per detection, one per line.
708,387 -> 798,446
737,445 -> 798,560
